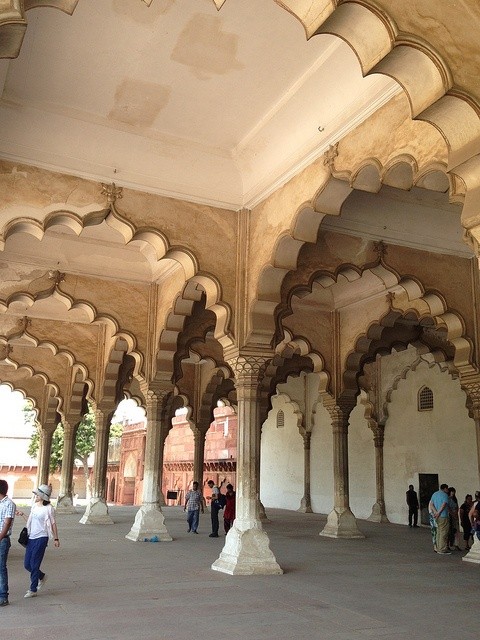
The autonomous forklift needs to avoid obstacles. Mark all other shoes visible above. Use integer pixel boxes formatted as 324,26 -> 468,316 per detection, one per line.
209,533 -> 219,537
438,550 -> 452,555
0,597 -> 9,606
187,528 -> 190,533
192,530 -> 198,534
407,524 -> 419,528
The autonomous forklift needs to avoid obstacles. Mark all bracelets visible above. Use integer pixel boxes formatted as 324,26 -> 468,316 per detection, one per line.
54,539 -> 59,541
210,497 -> 211,499
20,512 -> 24,516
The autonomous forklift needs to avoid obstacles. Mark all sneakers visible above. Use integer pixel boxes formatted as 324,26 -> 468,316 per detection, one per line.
38,574 -> 48,590
24,591 -> 38,598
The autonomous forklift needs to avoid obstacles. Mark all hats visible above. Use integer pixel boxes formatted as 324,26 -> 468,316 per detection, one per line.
32,484 -> 51,501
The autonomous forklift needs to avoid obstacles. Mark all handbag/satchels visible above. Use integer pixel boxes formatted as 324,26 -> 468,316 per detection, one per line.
18,527 -> 30,547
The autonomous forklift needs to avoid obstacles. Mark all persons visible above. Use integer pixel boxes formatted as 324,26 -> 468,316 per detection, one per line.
183,480 -> 236,537
406,483 -> 480,555
15,484 -> 61,599
0,479 -> 16,606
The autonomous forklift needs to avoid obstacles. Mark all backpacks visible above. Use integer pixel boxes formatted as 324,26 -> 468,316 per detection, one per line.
213,487 -> 226,509
